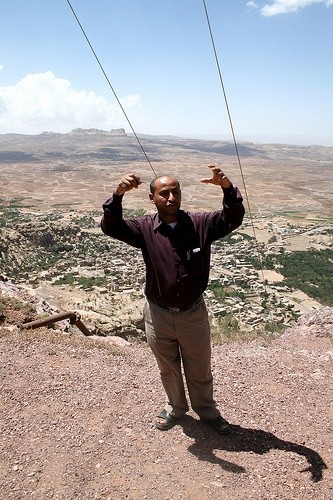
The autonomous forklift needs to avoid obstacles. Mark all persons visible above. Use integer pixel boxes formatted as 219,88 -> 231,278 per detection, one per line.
99,164 -> 246,435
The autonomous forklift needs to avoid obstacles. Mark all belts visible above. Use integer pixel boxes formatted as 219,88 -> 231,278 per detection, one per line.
147,294 -> 203,314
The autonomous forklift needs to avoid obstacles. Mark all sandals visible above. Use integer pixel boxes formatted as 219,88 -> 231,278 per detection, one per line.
156,410 -> 177,431
200,412 -> 232,435
